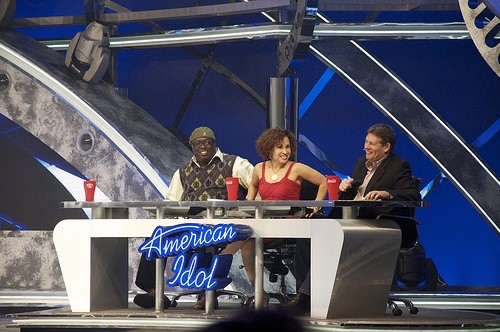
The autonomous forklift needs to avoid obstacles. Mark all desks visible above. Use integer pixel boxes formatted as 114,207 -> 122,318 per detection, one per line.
53,201 -> 421,319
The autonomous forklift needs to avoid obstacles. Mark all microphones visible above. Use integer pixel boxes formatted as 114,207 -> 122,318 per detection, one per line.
363,149 -> 366,150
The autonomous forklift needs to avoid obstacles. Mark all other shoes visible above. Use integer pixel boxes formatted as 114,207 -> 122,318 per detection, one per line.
272,293 -> 308,308
133,293 -> 171,310
193,298 -> 219,309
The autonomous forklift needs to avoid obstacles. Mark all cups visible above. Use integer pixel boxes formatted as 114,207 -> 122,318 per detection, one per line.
327,176 -> 340,200
225,177 -> 239,200
84,181 -> 96,202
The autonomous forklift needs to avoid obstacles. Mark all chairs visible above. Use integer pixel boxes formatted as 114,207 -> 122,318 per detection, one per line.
376,176 -> 421,316
242,175 -> 326,310
170,246 -> 248,307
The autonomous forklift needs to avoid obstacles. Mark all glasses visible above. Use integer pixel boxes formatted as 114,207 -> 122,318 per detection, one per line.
193,139 -> 213,147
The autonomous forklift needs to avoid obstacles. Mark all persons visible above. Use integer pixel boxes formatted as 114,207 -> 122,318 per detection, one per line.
218,127 -> 328,309
134,126 -> 255,310
275,122 -> 422,316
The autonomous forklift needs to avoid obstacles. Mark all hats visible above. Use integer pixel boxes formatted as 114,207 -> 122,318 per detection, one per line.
189,127 -> 216,143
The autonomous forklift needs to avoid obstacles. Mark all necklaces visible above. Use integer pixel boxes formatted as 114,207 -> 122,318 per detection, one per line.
270,163 -> 285,181
